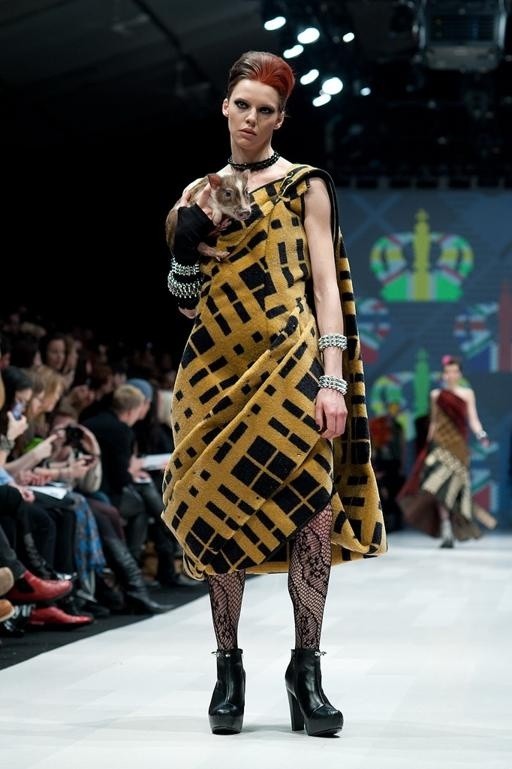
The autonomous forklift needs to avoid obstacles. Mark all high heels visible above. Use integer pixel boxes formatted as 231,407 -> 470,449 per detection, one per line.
208,649 -> 247,733
285,648 -> 344,736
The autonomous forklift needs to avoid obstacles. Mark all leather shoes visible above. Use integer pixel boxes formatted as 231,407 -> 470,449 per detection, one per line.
0,568 -> 201,630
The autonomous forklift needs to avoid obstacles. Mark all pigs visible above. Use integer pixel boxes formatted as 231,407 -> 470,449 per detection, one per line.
165,168 -> 253,262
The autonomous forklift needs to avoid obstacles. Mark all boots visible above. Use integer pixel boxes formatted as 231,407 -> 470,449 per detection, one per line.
439,519 -> 454,548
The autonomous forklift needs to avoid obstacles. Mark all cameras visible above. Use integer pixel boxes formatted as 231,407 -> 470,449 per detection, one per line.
63,424 -> 92,457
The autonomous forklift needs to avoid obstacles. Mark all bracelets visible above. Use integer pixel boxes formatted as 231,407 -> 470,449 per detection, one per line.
168,258 -> 200,278
166,272 -> 202,298
476,430 -> 488,440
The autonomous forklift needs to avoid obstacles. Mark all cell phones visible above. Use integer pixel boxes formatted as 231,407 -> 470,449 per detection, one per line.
11,400 -> 25,418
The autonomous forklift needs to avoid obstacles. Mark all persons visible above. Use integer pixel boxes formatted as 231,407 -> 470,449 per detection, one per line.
395,355 -> 500,549
163,47 -> 388,735
1,306 -> 430,634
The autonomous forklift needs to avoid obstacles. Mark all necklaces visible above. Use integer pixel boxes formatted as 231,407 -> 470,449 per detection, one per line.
228,152 -> 281,173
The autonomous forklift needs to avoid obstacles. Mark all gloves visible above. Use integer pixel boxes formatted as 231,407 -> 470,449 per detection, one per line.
171,205 -> 218,311
